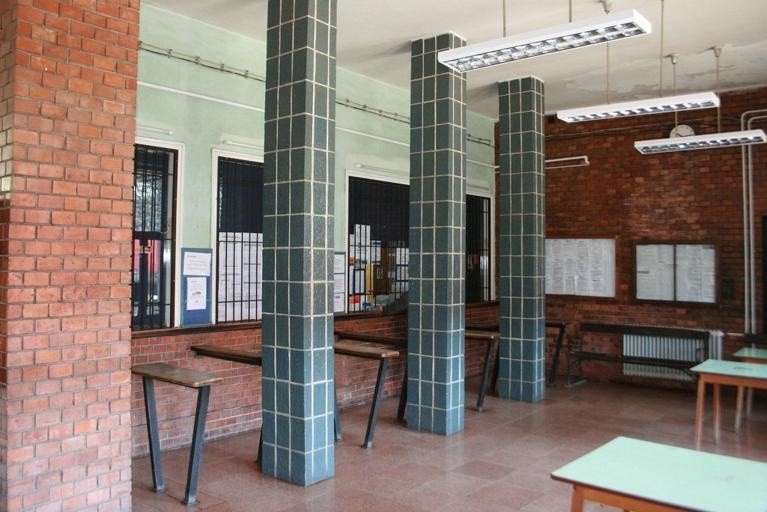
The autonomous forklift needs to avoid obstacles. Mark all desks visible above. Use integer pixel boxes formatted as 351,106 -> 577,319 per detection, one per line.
489,317 -> 567,397
733,348 -> 766,429
334,326 -> 408,422
549,434 -> 766,512
688,358 -> 766,450
188,343 -> 264,468
333,341 -> 399,451
461,329 -> 500,410
132,360 -> 221,505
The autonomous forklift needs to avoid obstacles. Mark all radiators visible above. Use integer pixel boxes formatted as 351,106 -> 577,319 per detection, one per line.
621,323 -> 723,383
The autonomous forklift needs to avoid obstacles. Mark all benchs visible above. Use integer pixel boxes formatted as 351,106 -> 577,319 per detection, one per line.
565,322 -> 709,392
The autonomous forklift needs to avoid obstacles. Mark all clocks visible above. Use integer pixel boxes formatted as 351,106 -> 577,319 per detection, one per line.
668,124 -> 696,142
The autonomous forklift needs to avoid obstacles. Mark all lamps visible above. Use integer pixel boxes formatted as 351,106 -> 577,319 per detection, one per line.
553,1 -> 721,127
635,46 -> 765,158
438,1 -> 655,78
